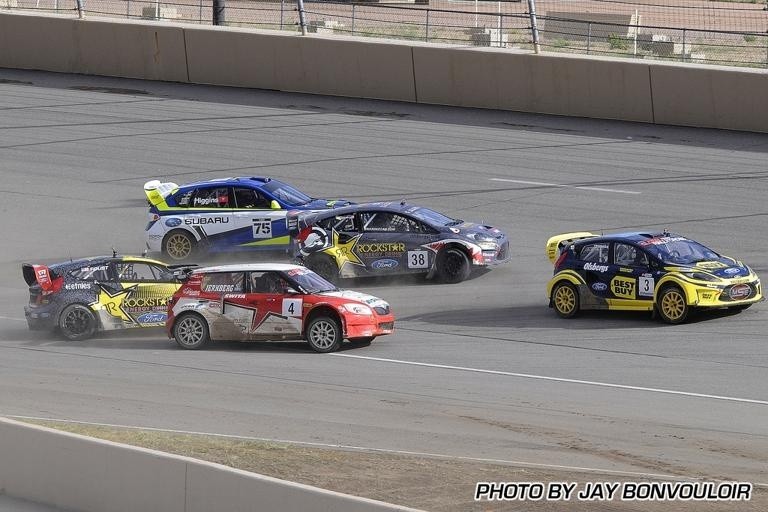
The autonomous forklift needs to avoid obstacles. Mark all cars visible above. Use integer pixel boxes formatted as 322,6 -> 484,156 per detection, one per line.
141,174 -> 359,262
165,263 -> 395,354
546,228 -> 763,324
22,250 -> 198,341
285,199 -> 512,284
